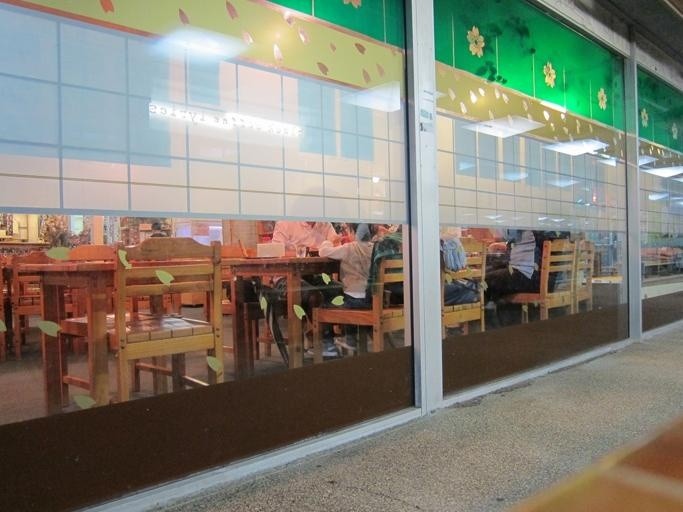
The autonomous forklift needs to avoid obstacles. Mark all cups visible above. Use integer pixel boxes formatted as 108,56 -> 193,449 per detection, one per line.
296,246 -> 306,258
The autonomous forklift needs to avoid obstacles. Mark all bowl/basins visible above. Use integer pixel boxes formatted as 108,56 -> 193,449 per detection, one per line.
309,251 -> 319,257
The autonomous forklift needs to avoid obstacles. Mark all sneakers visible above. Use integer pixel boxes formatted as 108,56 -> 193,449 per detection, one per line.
336,335 -> 358,352
308,346 -> 339,358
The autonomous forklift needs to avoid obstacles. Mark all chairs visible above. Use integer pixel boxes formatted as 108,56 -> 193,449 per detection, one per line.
1,237 -> 594,427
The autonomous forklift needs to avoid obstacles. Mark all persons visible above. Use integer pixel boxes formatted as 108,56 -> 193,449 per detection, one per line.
262,221 -> 573,351
148,222 -> 168,237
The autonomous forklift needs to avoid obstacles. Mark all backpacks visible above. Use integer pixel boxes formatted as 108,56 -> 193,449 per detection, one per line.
368,233 -> 403,296
